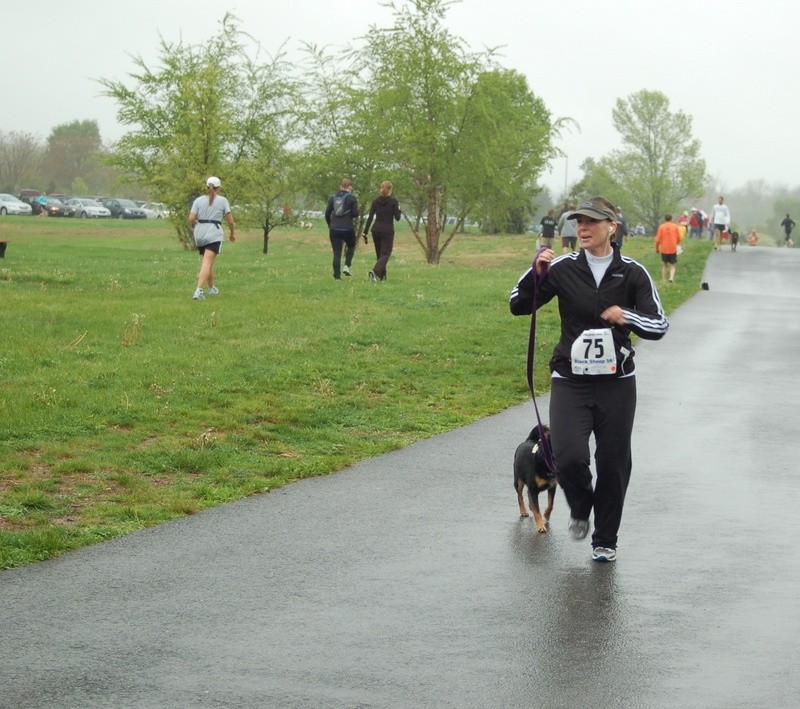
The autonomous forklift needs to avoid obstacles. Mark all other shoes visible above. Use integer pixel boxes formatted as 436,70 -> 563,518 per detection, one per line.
335,278 -> 341,282
369,270 -> 377,283
192,289 -> 206,301
208,286 -> 219,295
343,265 -> 352,276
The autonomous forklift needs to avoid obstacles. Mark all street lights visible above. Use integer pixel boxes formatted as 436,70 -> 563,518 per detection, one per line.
564,154 -> 568,200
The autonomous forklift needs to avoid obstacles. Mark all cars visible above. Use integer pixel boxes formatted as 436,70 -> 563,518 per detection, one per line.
357,213 -> 480,227
103,199 -> 179,219
229,203 -> 327,218
0,193 -> 32,215
19,189 -> 112,219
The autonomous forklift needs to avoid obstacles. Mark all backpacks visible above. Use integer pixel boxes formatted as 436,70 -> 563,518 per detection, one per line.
327,193 -> 351,217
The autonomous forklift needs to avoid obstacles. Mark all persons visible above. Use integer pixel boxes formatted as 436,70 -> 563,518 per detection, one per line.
189,177 -> 236,300
538,195 -> 795,255
507,198 -> 669,562
324,178 -> 361,280
655,213 -> 682,283
362,181 -> 400,282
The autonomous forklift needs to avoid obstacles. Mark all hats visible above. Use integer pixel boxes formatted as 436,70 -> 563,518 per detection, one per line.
207,176 -> 221,187
566,200 -> 618,222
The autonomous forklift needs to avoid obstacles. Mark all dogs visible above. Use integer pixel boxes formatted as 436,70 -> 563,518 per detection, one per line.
513,424 -> 558,533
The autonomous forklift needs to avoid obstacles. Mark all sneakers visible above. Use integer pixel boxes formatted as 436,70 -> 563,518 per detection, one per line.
568,518 -> 589,539
592,546 -> 616,561
